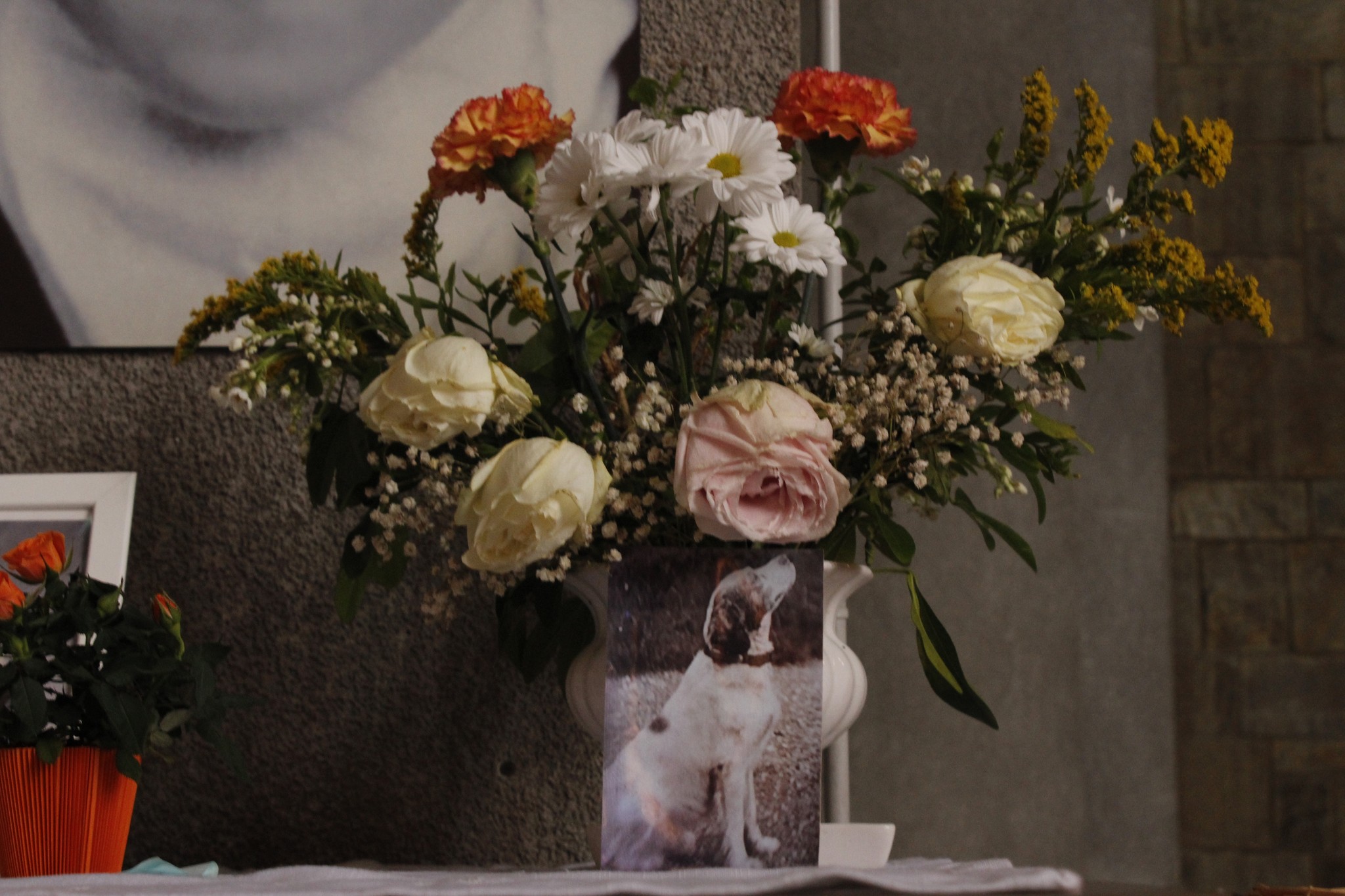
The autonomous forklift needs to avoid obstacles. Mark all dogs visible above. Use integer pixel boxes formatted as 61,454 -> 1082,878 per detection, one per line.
601,549 -> 804,872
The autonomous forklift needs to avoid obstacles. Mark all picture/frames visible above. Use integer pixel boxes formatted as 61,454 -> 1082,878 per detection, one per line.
0,472 -> 138,701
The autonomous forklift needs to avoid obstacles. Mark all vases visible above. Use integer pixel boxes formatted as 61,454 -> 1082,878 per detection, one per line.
0,746 -> 143,878
564,558 -> 876,822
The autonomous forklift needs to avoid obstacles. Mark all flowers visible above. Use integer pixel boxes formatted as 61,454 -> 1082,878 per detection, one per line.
167,67 -> 1275,733
0,530 -> 261,789
